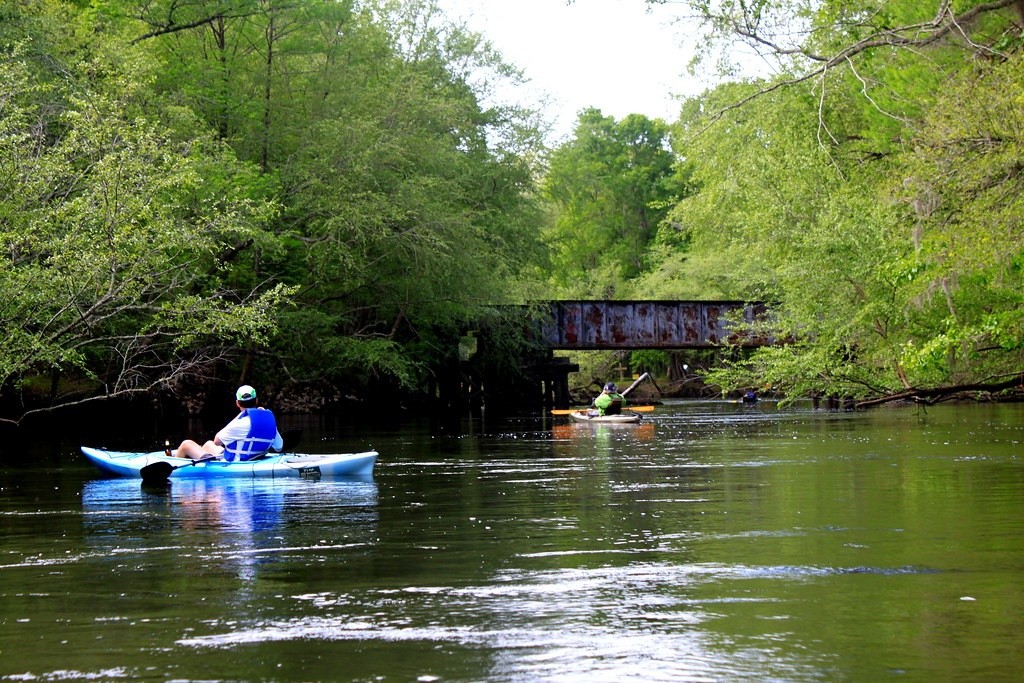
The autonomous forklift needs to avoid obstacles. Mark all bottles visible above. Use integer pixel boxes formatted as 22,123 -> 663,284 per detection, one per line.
165,440 -> 171,456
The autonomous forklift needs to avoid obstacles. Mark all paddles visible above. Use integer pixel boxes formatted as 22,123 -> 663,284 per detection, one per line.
551,404 -> 657,415
139,453 -> 226,482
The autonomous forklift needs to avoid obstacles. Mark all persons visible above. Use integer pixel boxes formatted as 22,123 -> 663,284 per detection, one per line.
594,383 -> 626,416
175,385 -> 283,462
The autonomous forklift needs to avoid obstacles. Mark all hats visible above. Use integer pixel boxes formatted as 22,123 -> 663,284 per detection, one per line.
236,385 -> 257,401
604,382 -> 618,393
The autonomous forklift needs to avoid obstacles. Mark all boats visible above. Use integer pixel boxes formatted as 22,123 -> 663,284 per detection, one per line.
78,445 -> 381,479
571,409 -> 642,424
